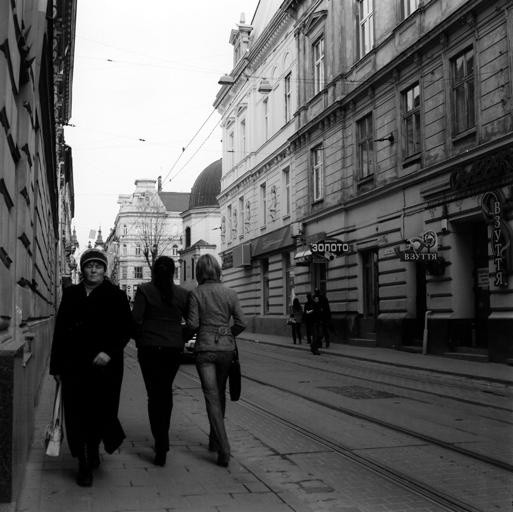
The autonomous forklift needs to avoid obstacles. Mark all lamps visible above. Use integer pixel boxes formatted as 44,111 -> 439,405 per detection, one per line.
217,73 -> 235,85
257,77 -> 273,93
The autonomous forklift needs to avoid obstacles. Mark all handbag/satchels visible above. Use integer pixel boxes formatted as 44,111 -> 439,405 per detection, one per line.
43,418 -> 64,458
286,314 -> 296,325
226,358 -> 244,404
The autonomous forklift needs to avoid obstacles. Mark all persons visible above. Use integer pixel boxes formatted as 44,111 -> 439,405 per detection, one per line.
131,257 -> 196,467
47,248 -> 132,488
286,286 -> 335,356
181,255 -> 248,468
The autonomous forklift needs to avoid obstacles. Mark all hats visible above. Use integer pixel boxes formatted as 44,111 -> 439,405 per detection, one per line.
79,248 -> 109,272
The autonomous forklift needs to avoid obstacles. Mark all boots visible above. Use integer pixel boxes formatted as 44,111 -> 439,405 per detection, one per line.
75,439 -> 101,488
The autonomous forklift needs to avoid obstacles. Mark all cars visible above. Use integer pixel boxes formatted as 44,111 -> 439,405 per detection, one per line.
182,321 -> 197,358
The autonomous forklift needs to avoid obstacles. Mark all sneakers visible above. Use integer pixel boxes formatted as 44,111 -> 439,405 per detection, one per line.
216,450 -> 231,467
208,437 -> 217,452
310,347 -> 320,355
152,442 -> 170,465
